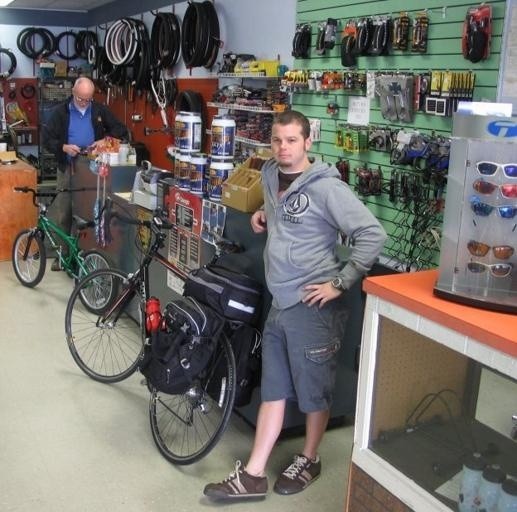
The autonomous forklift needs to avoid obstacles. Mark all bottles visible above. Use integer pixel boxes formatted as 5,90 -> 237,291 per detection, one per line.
173,110 -> 208,195
455,448 -> 487,512
207,161 -> 235,202
210,114 -> 236,161
477,464 -> 506,512
146,295 -> 161,335
119,137 -> 129,166
498,478 -> 517,512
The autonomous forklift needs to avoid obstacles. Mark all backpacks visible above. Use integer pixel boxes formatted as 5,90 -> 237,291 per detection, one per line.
138,295 -> 216,394
182,262 -> 268,320
207,320 -> 259,406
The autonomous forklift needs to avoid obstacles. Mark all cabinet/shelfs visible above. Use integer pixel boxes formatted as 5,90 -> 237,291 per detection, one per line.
206,71 -> 283,148
0,155 -> 39,263
345,269 -> 517,512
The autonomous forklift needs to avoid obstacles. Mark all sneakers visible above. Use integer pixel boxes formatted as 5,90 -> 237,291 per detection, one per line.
202,468 -> 268,499
273,452 -> 326,496
51,256 -> 63,271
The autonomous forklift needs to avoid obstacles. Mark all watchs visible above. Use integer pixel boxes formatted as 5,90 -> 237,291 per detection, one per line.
332,277 -> 345,293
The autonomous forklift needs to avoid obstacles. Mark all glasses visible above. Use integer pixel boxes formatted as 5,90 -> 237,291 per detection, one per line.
75,95 -> 95,104
470,180 -> 517,199
468,201 -> 517,219
465,239 -> 516,261
467,263 -> 516,279
474,159 -> 517,179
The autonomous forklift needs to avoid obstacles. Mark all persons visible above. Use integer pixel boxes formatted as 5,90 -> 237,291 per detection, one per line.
49,78 -> 127,271
202,110 -> 386,498
